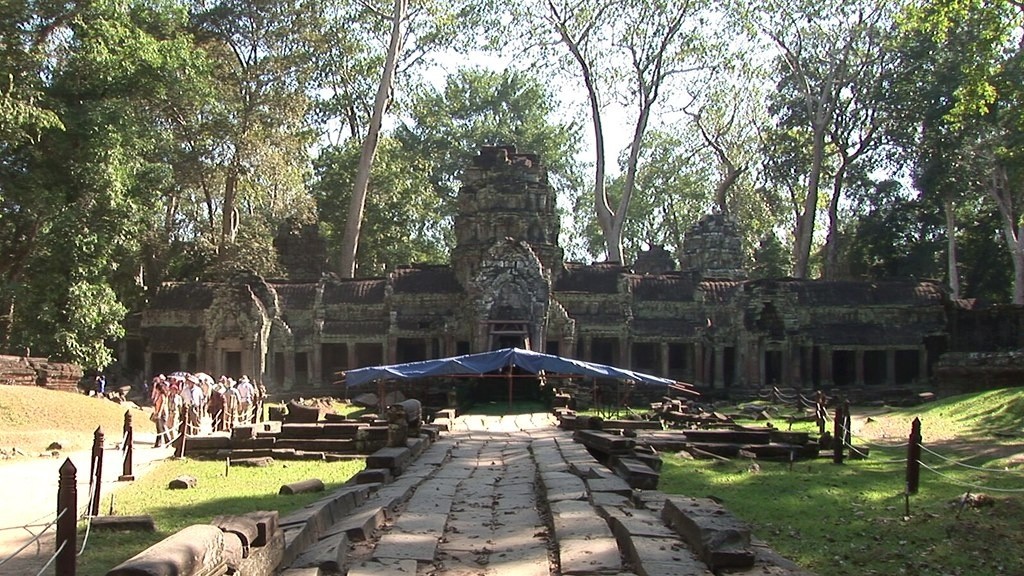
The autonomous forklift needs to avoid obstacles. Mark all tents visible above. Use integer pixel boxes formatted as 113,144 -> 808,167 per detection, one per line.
332,347 -> 700,414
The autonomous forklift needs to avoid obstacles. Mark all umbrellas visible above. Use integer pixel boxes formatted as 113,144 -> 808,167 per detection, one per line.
167,372 -> 191,380
194,373 -> 214,384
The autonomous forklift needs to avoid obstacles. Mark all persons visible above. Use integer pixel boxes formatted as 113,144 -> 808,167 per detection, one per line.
151,374 -> 257,448
97,375 -> 105,394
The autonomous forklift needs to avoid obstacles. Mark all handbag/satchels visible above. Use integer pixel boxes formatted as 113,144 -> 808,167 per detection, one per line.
168,413 -> 176,428
230,389 -> 238,409
150,412 -> 155,422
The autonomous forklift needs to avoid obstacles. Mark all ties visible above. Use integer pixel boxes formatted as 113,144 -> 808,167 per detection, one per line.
190,389 -> 194,403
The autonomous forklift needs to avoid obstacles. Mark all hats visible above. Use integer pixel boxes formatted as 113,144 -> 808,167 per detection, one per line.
213,383 -> 226,393
164,381 -> 170,386
224,378 -> 237,387
239,375 -> 250,383
187,375 -> 199,384
171,385 -> 178,389
160,374 -> 166,380
